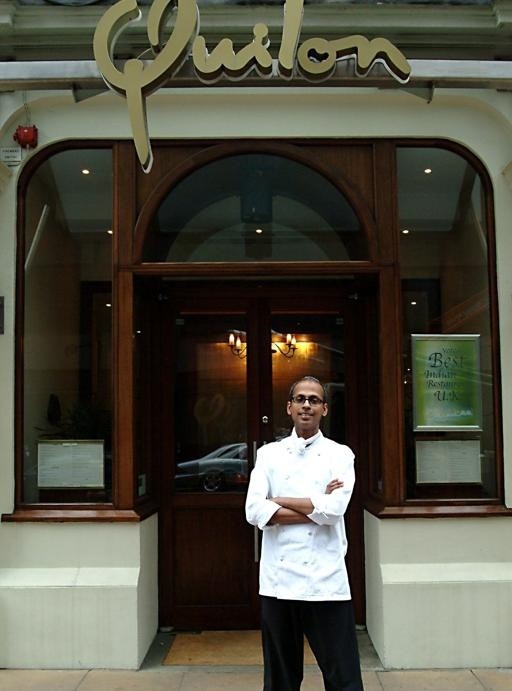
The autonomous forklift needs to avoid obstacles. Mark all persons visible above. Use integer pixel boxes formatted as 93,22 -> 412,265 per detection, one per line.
245,374 -> 363,690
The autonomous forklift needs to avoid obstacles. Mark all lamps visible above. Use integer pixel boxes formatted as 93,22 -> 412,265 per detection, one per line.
228,330 -> 299,362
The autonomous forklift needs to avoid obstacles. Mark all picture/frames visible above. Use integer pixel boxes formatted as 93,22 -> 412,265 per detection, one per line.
409,332 -> 486,435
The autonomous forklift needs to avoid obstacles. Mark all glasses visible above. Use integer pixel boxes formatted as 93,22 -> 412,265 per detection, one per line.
291,396 -> 324,407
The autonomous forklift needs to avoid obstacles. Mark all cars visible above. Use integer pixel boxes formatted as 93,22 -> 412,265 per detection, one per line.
176,443 -> 248,492
